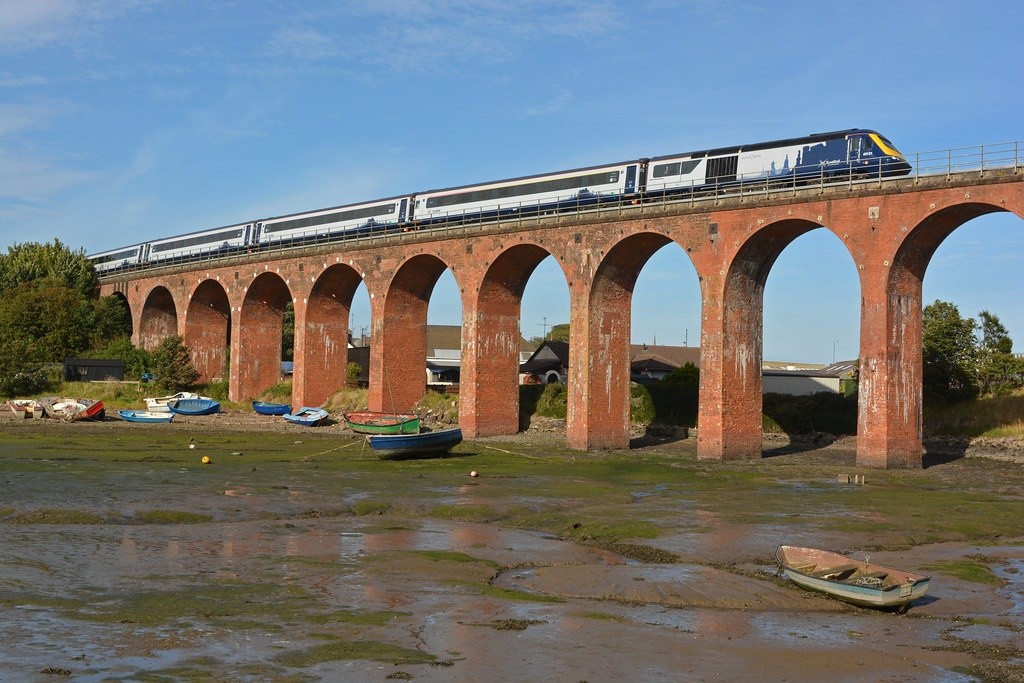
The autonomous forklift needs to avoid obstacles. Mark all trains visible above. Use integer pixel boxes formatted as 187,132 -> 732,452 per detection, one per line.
85,128 -> 912,278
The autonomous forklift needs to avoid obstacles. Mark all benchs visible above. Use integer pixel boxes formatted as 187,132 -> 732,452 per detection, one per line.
291,409 -> 309,416
837,570 -> 889,584
811,564 -> 859,578
126,412 -> 135,417
878,582 -> 899,591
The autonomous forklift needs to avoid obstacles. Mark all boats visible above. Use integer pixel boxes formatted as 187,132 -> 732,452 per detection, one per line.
144,391 -> 213,412
8,399 -> 43,419
366,427 -> 464,460
251,400 -> 293,415
776,543 -> 931,607
342,410 -> 421,433
41,396 -> 108,422
118,409 -> 176,422
166,399 -> 221,414
283,406 -> 330,427
